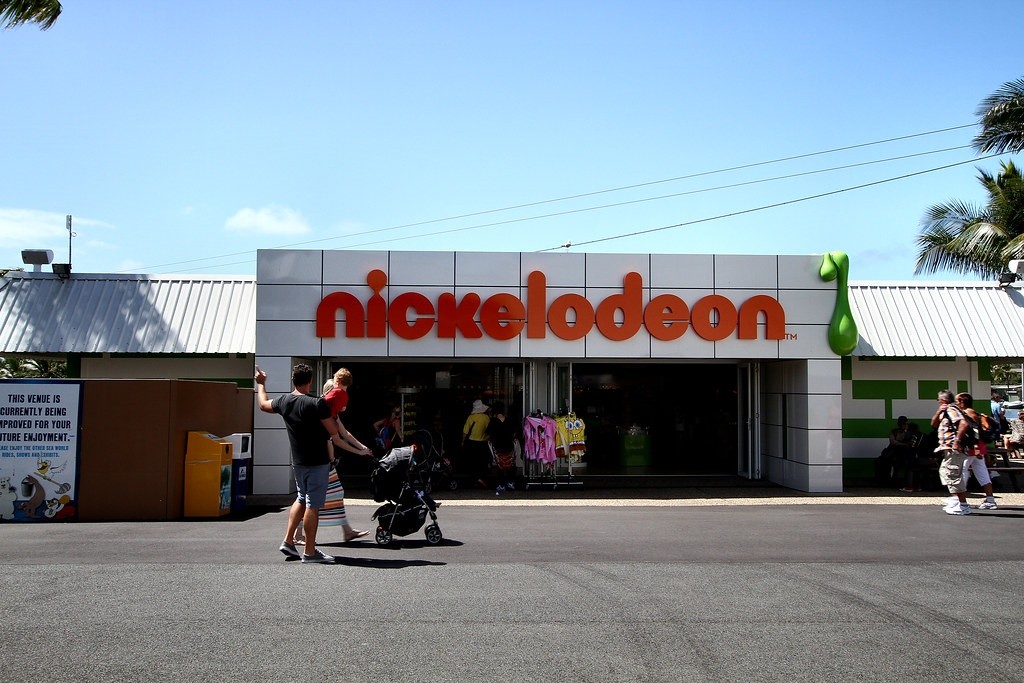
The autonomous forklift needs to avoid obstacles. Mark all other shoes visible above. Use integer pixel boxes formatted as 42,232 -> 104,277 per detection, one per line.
496,485 -> 506,492
302,549 -> 340,564
914,488 -> 922,492
279,542 -> 301,558
344,529 -> 371,544
507,482 -> 517,491
898,486 -> 914,493
292,536 -> 318,547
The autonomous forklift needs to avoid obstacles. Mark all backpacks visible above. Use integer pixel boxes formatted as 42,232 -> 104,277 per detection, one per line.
996,407 -> 1012,434
375,419 -> 397,455
962,409 -> 1001,443
944,403 -> 980,449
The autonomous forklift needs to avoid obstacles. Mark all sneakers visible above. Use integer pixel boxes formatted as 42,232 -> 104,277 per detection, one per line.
945,504 -> 973,515
942,500 -> 959,510
975,500 -> 998,510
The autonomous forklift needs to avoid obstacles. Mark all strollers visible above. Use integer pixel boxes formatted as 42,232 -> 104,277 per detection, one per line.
368,444 -> 444,548
407,427 -> 459,495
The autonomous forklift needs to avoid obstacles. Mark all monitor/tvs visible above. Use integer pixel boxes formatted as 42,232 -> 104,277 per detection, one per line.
223,434 -> 251,460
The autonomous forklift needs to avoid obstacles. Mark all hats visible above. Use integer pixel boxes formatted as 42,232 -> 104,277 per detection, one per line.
897,415 -> 909,424
471,399 -> 489,414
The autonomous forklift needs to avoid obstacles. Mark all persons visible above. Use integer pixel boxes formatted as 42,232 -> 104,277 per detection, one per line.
254,364 -> 339,563
890,390 -> 1024,515
323,369 -> 353,472
293,378 -> 373,546
374,400 -> 517,493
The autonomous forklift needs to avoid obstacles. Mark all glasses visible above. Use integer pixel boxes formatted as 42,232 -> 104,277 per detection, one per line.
936,398 -> 940,401
994,395 -> 1001,399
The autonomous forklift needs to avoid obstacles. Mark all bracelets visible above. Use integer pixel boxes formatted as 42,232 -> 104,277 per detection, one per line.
955,438 -> 961,443
